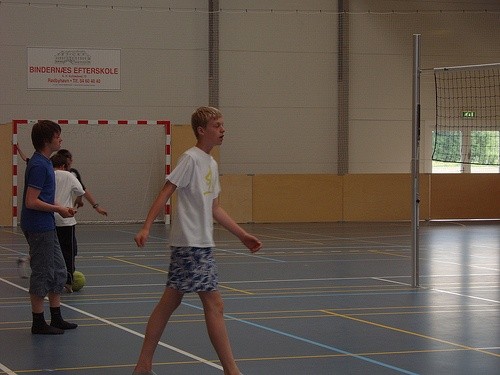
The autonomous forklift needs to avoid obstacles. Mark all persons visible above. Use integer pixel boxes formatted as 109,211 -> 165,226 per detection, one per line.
131,105 -> 264,375
14,143 -> 109,293
20,120 -> 79,334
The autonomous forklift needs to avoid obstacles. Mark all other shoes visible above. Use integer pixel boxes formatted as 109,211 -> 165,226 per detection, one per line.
63,285 -> 73,292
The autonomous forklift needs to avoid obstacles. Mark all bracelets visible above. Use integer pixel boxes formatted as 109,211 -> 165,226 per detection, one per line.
93,203 -> 99,208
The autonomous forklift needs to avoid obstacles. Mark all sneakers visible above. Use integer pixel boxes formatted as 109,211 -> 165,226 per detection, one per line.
32,319 -> 64,335
50,316 -> 77,329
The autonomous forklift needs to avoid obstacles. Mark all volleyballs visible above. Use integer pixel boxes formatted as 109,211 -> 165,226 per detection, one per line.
72,271 -> 86,292
